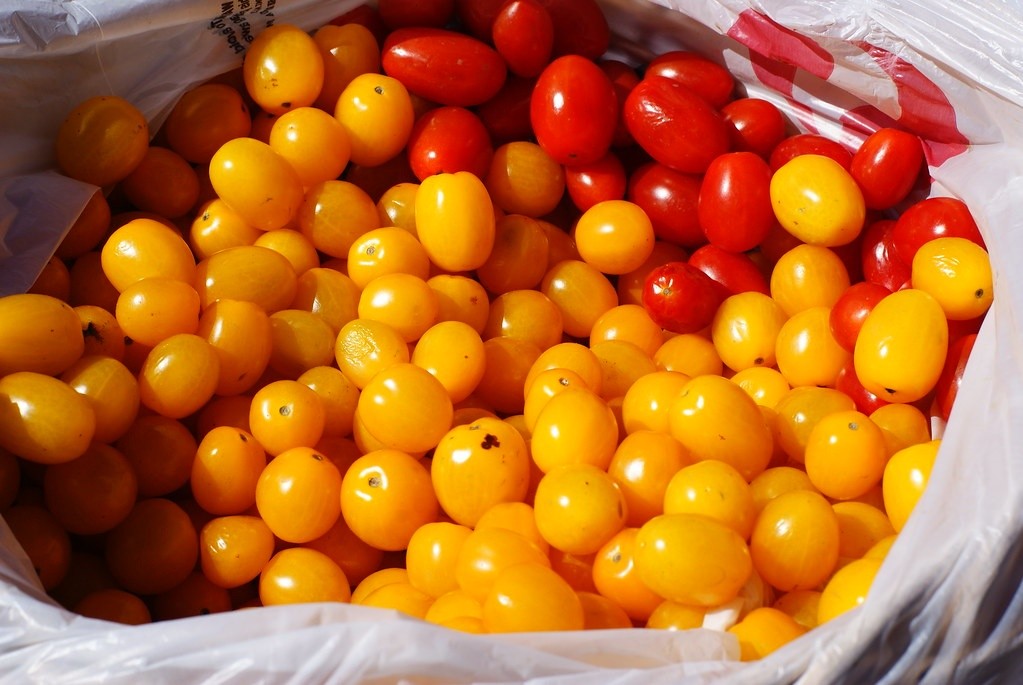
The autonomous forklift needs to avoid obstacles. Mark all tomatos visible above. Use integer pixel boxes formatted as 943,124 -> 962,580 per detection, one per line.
0,0 -> 994,661
